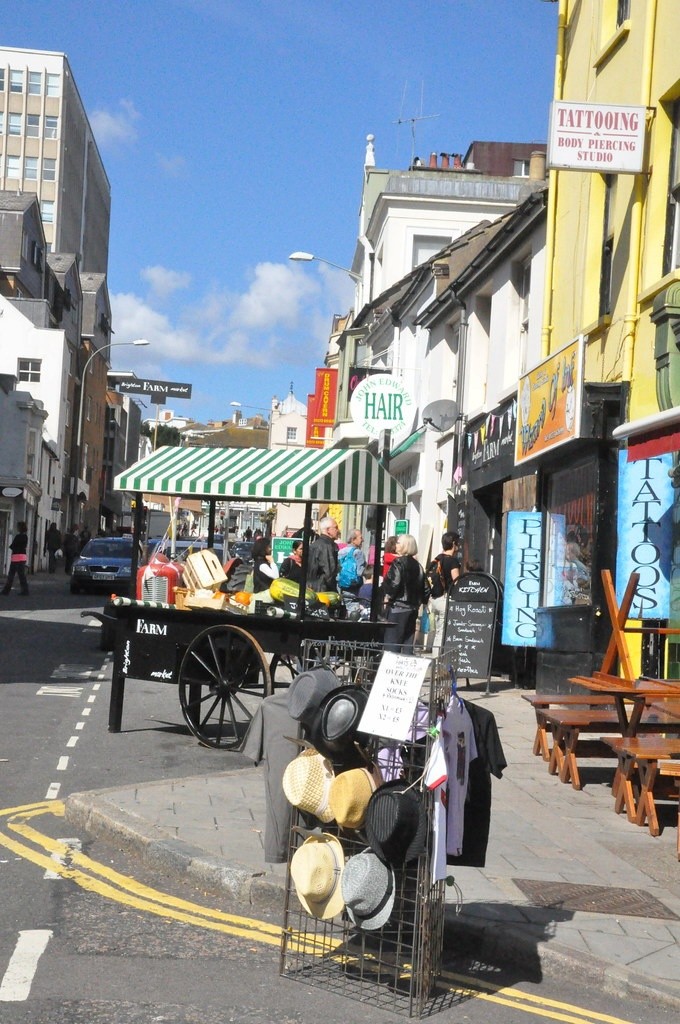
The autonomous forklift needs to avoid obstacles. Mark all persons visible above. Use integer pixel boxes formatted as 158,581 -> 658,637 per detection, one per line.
0,522 -> 28,595
228,519 -> 483,660
44,523 -> 125,574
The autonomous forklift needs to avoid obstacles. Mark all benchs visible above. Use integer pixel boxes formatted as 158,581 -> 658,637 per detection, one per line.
520,693 -> 680,862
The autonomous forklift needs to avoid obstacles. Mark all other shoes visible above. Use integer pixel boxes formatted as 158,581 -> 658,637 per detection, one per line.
0,590 -> 9,595
18,591 -> 30,596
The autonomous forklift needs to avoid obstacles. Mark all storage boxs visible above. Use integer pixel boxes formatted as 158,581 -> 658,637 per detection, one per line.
535,651 -> 594,695
532,603 -> 594,652
184,590 -> 248,617
181,546 -> 228,590
254,600 -> 284,615
171,586 -> 193,610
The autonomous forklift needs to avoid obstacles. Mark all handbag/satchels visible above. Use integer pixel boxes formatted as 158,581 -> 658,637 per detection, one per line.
420,607 -> 430,633
54,549 -> 64,560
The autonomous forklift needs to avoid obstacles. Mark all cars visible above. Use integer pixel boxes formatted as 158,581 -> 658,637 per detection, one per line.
70,537 -> 143,595
150,541 -> 232,567
140,539 -> 159,566
229,542 -> 254,569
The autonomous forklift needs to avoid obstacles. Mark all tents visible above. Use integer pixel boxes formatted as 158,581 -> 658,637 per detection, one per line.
114,444 -> 408,616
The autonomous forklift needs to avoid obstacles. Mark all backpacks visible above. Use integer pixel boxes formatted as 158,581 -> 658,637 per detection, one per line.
425,556 -> 446,598
338,547 -> 363,589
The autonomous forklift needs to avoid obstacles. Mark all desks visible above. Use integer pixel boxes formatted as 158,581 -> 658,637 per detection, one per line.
567,568 -> 680,693
593,692 -> 680,802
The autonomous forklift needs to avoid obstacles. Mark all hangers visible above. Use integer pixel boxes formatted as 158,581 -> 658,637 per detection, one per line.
439,687 -> 447,719
448,664 -> 464,714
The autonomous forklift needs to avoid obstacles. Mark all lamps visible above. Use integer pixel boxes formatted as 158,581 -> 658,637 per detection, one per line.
289,251 -> 364,286
434,460 -> 443,472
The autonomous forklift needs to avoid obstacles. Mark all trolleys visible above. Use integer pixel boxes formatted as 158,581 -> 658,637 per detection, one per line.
80,448 -> 408,751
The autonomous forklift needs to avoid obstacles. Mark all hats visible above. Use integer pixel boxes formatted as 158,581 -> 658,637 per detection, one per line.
283,668 -> 426,931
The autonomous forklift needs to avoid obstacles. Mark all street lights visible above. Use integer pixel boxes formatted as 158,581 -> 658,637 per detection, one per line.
71,338 -> 149,535
